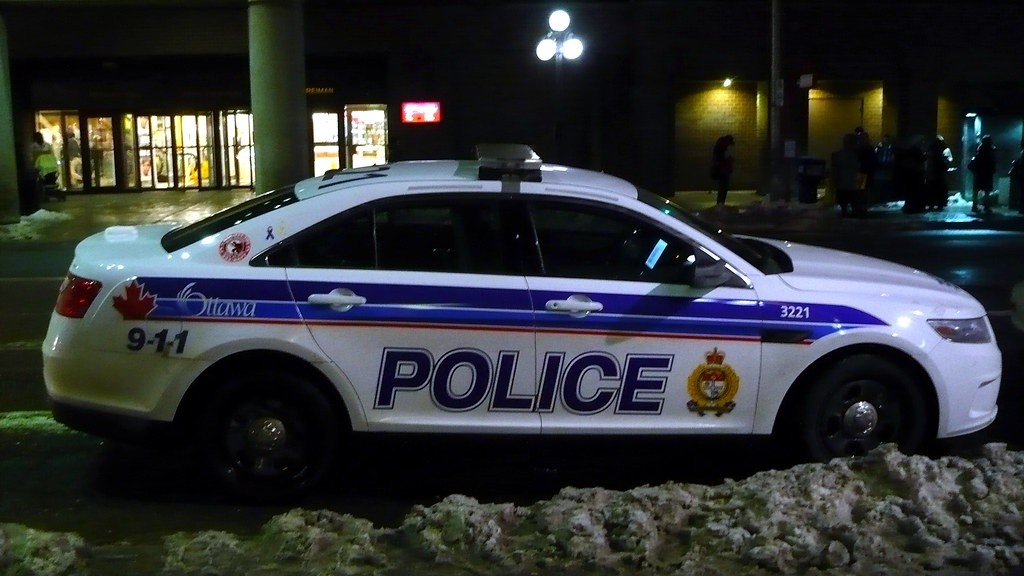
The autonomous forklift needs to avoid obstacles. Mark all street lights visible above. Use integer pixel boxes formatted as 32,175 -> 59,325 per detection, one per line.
536,10 -> 584,161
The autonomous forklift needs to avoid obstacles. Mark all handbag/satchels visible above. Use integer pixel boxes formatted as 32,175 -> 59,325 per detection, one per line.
967,151 -> 981,172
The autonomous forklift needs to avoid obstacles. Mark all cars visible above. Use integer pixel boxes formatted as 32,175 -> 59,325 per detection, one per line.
42,142 -> 1002,501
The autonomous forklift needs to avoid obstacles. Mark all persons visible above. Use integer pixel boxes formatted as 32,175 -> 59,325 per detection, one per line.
27,134 -> 51,172
831,127 -> 953,214
50,132 -> 82,187
708,135 -> 735,205
1006,137 -> 1024,214
967,135 -> 998,213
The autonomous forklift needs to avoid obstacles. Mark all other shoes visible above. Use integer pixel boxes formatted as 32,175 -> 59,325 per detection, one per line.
971,206 -> 981,212
984,207 -> 993,213
